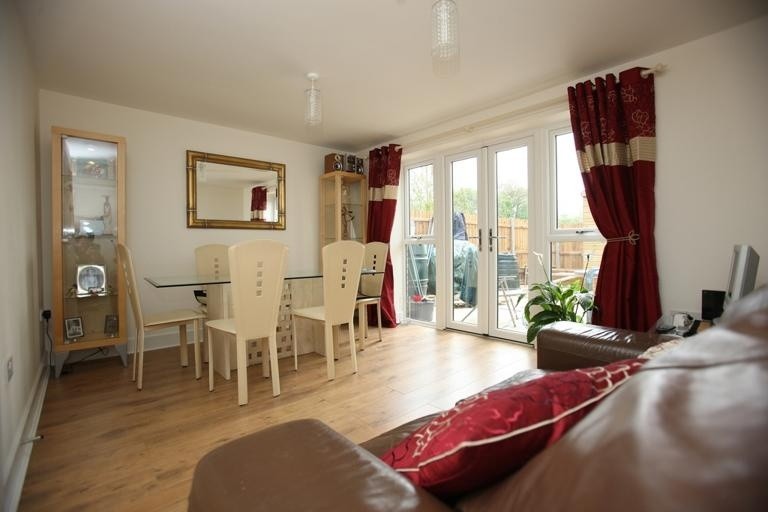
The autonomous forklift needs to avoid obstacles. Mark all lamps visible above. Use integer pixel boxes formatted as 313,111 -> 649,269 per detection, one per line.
303,73 -> 322,128
431,0 -> 461,60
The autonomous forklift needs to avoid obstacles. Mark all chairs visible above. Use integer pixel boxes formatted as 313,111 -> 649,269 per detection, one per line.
290,241 -> 361,381
354,240 -> 389,351
192,243 -> 238,278
461,253 -> 528,328
584,269 -> 601,324
203,242 -> 287,404
121,247 -> 207,392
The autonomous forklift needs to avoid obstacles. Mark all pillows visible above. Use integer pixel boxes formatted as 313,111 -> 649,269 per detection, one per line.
377,359 -> 652,501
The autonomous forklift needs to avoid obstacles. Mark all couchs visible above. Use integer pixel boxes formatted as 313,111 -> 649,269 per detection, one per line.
188,288 -> 768,512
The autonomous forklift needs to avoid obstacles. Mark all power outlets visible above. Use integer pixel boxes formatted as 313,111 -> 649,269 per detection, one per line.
40,309 -> 45,322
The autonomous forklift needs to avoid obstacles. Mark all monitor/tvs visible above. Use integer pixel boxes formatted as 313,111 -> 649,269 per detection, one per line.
712,245 -> 760,325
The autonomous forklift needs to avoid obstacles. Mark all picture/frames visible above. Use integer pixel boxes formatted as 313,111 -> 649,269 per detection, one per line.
104,315 -> 119,333
64,317 -> 84,339
74,263 -> 106,294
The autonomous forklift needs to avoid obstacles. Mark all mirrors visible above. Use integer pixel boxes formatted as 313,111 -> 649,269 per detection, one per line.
186,150 -> 286,230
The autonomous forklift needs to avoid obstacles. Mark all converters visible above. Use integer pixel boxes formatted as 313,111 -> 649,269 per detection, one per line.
43,309 -> 51,320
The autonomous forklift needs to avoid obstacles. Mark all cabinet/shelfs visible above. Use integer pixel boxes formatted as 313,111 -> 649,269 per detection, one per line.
51,125 -> 128,379
319,170 -> 368,272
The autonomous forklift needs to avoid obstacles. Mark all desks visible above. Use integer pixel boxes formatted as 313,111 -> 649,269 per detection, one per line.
647,313 -> 712,338
140,265 -> 388,381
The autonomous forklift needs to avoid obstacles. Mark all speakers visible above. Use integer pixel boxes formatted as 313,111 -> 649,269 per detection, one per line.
325,153 -> 343,173
355,158 -> 364,174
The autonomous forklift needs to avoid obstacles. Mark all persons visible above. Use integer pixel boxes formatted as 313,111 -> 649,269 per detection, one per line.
82,268 -> 98,290
